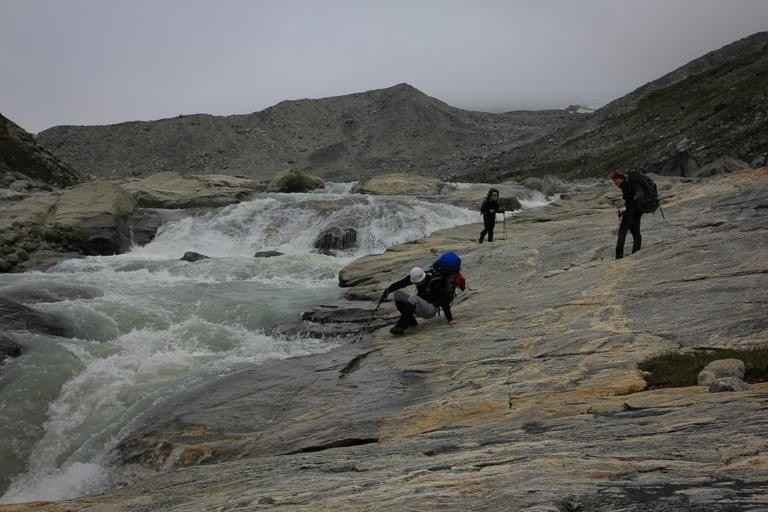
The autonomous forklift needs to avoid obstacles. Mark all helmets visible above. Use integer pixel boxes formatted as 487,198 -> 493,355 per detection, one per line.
410,267 -> 426,283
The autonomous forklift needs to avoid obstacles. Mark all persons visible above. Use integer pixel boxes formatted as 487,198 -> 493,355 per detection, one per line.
381,267 -> 459,334
611,170 -> 641,259
479,189 -> 505,243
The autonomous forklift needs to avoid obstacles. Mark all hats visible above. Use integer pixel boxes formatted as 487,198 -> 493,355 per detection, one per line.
612,170 -> 625,178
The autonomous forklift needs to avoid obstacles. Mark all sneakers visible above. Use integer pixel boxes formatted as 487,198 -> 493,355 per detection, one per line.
398,319 -> 417,326
390,324 -> 407,335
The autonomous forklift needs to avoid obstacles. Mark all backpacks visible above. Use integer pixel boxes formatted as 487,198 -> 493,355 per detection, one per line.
432,252 -> 460,304
628,167 -> 660,213
